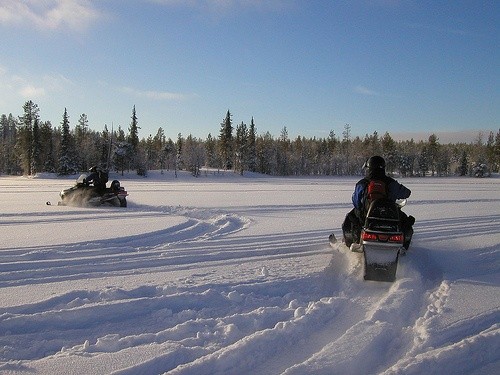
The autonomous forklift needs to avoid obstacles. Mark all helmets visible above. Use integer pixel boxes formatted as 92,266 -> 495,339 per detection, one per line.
88,166 -> 97,172
362,156 -> 384,168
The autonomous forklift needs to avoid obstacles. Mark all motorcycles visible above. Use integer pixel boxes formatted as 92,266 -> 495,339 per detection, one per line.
328,197 -> 415,283
46,178 -> 129,207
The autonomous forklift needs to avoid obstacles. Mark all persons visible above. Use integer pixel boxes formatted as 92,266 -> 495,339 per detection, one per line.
351,156 -> 414,250
86,166 -> 106,190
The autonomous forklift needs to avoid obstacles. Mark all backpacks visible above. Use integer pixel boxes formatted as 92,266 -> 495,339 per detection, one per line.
364,177 -> 391,209
97,170 -> 108,183
